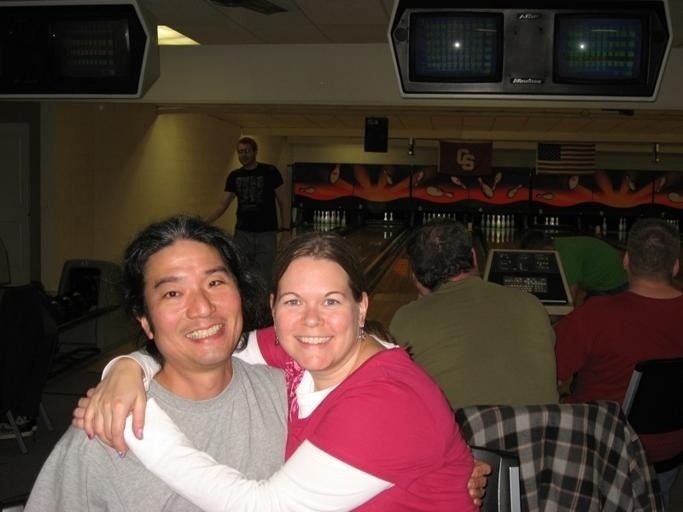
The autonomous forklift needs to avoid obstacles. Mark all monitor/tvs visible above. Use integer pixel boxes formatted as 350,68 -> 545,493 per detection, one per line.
553,12 -> 646,87
22,17 -> 131,94
405,9 -> 506,86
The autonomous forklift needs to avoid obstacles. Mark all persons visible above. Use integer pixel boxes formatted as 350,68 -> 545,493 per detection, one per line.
72,230 -> 479,512
524,229 -> 629,309
206,137 -> 287,280
554,218 -> 683,504
23,216 -> 492,512
388,218 -> 557,409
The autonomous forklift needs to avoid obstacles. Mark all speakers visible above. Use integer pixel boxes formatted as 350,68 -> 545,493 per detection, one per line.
364,116 -> 389,154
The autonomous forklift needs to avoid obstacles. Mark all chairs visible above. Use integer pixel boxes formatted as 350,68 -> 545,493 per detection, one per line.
621,357 -> 683,473
0,281 -> 60,456
466,443 -> 521,511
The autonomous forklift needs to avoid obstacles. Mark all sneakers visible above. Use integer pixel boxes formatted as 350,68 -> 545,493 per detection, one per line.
1,416 -> 37,441
